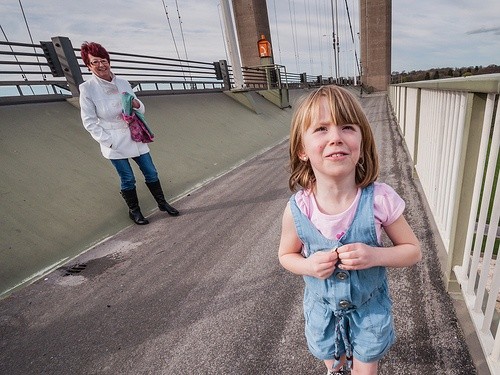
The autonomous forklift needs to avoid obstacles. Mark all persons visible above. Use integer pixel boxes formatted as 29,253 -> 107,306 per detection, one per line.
278,85 -> 422,375
79,42 -> 180,225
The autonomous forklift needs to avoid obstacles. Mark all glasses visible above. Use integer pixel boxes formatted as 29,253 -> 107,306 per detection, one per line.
89,60 -> 108,65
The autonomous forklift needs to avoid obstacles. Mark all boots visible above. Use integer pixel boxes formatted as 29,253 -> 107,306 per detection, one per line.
120,186 -> 149,225
145,179 -> 179,215
